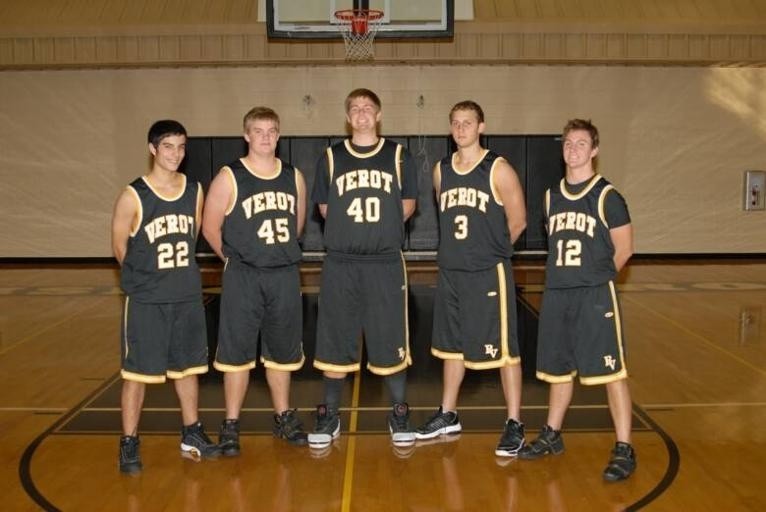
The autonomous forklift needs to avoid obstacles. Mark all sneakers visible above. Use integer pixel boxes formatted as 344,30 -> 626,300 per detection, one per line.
220,420 -> 240,458
496,419 -> 527,456
272,409 -> 308,445
388,404 -> 416,446
308,405 -> 341,448
604,443 -> 636,481
414,404 -> 462,440
118,436 -> 143,474
519,425 -> 564,459
180,418 -> 221,460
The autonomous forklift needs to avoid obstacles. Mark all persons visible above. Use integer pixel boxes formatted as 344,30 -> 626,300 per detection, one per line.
420,101 -> 527,456
307,89 -> 416,443
202,105 -> 310,457
112,118 -> 222,475
518,118 -> 636,483
